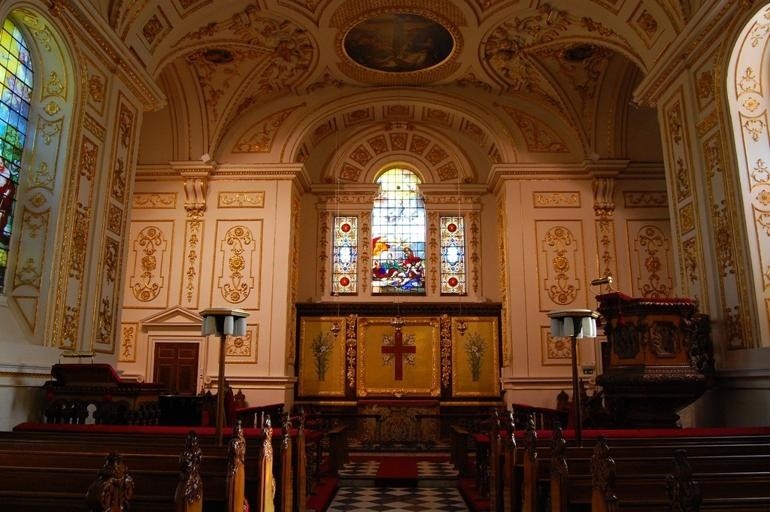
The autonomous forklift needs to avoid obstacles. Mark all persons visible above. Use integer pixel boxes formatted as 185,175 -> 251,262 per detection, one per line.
0,156 -> 17,237
355,19 -> 438,68
374,247 -> 424,291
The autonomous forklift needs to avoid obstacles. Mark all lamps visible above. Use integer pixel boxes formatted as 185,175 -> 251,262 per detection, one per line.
198,307 -> 252,446
544,305 -> 605,443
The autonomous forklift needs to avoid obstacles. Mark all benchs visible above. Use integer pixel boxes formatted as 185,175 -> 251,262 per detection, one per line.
472,407 -> 770,512
1,406 -> 347,512
